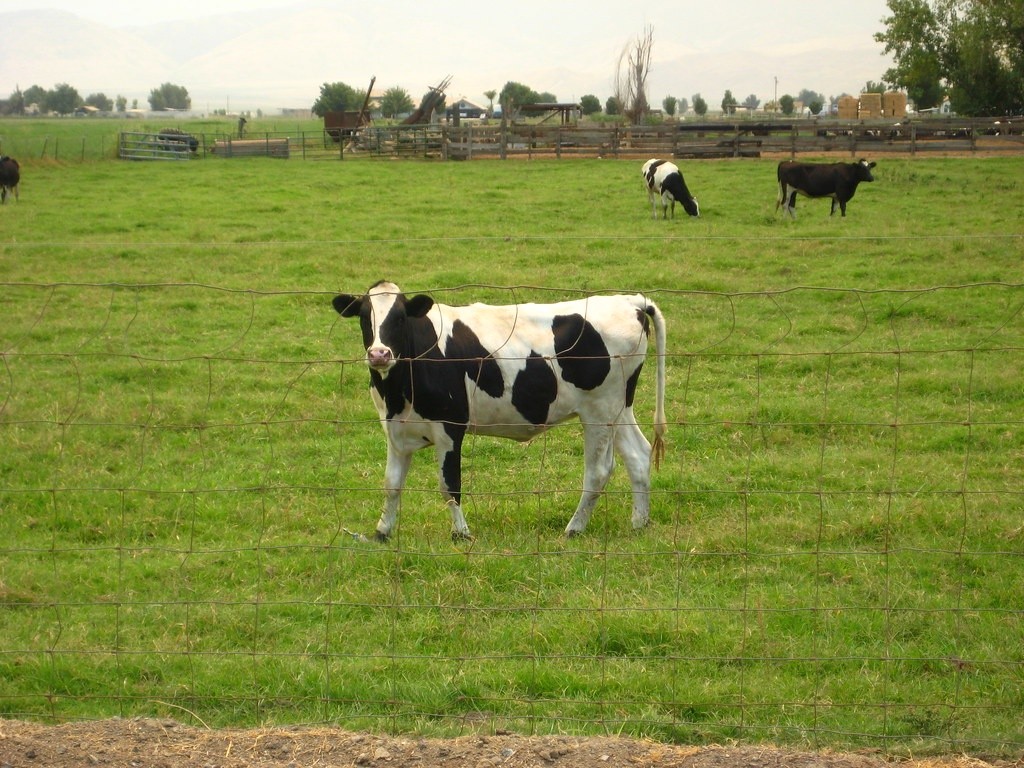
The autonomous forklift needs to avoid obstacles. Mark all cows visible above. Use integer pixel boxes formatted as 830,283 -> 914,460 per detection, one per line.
816,121 -> 1009,136
774,158 -> 876,218
641,158 -> 699,221
0,156 -> 20,204
158,128 -> 197,153
332,280 -> 666,542
